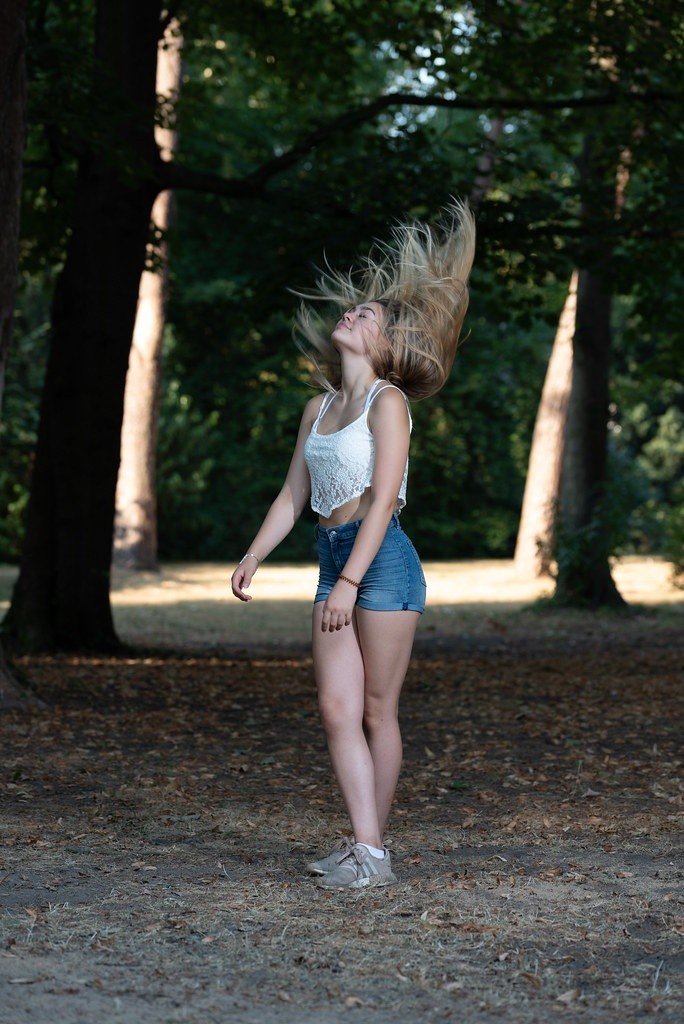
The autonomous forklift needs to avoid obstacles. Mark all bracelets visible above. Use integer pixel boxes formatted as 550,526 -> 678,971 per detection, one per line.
337,574 -> 363,588
238,553 -> 259,564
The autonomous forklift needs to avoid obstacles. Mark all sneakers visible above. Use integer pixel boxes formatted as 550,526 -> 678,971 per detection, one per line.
317,844 -> 396,891
307,837 -> 355,875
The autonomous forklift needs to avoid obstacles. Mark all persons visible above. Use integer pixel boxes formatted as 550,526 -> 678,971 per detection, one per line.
232,194 -> 478,890
